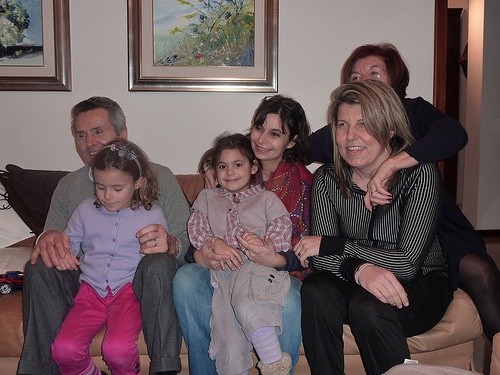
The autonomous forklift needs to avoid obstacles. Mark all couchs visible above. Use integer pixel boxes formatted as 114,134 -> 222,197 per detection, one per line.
0,174 -> 484,375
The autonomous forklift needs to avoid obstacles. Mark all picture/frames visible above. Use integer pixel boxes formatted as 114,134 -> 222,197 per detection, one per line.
0,0 -> 72,93
126,0 -> 279,93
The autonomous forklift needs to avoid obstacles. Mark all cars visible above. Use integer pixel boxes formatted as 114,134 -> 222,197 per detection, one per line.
0,270 -> 24,295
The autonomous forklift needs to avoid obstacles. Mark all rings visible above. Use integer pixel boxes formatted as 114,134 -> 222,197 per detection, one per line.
244,249 -> 248,254
371,190 -> 377,194
298,252 -> 302,257
154,239 -> 157,247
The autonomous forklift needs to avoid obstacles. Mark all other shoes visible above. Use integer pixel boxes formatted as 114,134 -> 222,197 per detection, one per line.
259,353 -> 294,375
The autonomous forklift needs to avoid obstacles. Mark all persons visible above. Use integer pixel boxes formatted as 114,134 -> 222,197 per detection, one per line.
187,134 -> 292,375
49,137 -> 167,375
16,97 -> 192,375
294,80 -> 452,375
204,43 -> 500,344
172,95 -> 313,375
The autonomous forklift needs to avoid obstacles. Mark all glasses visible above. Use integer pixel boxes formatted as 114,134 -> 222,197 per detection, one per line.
349,69 -> 387,82
